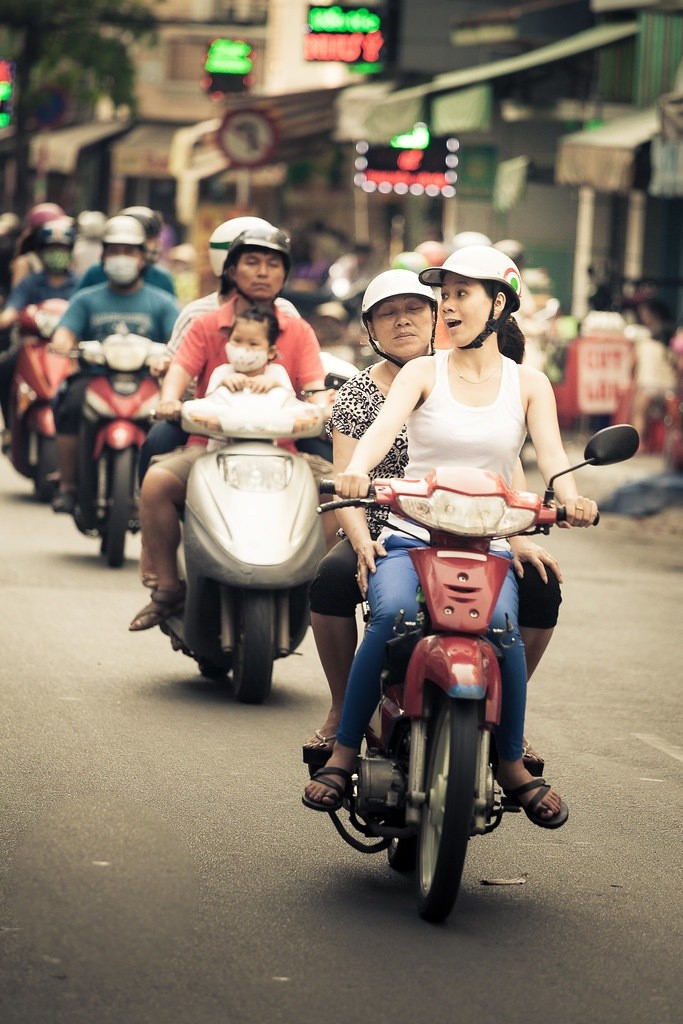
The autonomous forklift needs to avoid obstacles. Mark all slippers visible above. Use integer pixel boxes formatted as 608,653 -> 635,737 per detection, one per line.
496,772 -> 569,829
301,767 -> 353,812
519,736 -> 544,768
303,729 -> 337,750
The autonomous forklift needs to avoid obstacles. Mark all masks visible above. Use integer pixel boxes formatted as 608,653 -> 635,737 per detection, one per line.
103,255 -> 140,286
45,251 -> 72,273
224,342 -> 272,373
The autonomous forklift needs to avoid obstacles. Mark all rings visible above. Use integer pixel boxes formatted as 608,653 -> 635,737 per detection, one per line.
577,506 -> 585,513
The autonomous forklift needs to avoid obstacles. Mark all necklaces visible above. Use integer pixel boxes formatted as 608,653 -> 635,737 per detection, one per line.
449,350 -> 501,384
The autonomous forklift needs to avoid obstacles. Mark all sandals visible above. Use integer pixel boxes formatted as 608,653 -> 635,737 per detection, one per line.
129,580 -> 186,631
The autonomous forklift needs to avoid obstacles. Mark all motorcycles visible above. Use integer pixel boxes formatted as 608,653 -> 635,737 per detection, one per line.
309,422 -> 640,925
1,298 -> 78,502
41,329 -> 174,569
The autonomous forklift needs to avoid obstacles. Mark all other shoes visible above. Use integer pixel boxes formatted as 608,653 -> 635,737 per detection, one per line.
46,468 -> 62,482
54,493 -> 76,513
138,564 -> 160,588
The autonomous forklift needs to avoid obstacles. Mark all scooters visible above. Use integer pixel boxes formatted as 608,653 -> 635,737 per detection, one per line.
148,372 -> 354,703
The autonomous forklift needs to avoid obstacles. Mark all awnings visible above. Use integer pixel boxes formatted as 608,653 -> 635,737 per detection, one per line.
27,22 -> 683,199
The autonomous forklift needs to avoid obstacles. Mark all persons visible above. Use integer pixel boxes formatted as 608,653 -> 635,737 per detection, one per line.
0,198 -> 682,830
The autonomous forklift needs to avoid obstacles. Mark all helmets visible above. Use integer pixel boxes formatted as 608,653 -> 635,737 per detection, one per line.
118,206 -> 163,235
101,216 -> 147,251
209,217 -> 274,277
361,269 -> 438,320
418,244 -> 522,313
37,220 -> 74,249
28,202 -> 64,231
228,228 -> 292,280
